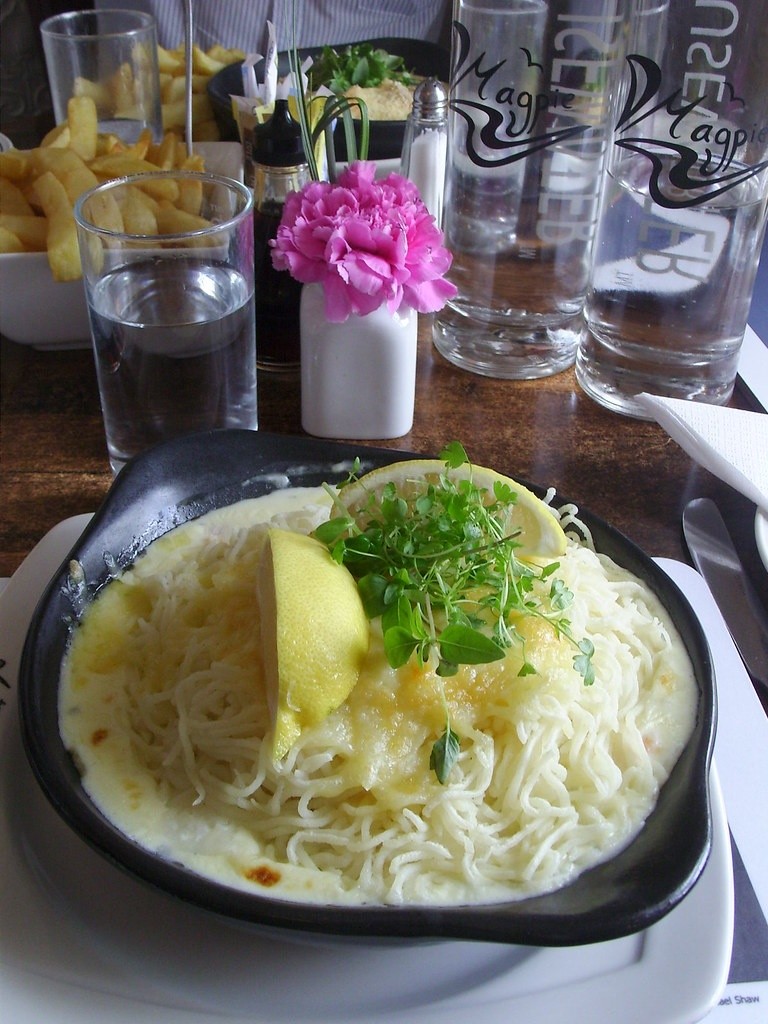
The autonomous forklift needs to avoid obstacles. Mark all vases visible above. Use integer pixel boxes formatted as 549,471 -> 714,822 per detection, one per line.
287,283 -> 419,437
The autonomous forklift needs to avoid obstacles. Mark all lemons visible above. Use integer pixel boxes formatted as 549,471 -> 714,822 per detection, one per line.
324,458 -> 571,561
254,528 -> 374,767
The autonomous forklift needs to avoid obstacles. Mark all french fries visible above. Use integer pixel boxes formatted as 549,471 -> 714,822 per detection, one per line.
0,93 -> 234,280
75,36 -> 249,140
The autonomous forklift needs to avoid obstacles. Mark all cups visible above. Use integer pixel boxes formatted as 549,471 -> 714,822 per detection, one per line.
40,6 -> 164,146
575,1 -> 768,421
429,0 -> 636,379
73,170 -> 261,480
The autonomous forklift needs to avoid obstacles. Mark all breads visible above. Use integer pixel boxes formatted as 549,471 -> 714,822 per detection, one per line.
339,78 -> 415,121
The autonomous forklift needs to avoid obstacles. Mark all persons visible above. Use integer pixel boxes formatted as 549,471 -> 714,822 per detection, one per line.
96,0 -> 454,56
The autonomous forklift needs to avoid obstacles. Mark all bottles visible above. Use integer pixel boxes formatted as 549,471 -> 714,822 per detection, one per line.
249,100 -> 317,375
395,75 -> 449,241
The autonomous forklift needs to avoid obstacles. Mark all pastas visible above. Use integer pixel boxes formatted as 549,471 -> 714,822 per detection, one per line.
122,504 -> 672,907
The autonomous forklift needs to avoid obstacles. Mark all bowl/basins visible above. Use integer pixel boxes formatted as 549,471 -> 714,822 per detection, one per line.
0,139 -> 248,353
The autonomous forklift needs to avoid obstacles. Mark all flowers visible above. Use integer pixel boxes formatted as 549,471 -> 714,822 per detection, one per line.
270,160 -> 461,321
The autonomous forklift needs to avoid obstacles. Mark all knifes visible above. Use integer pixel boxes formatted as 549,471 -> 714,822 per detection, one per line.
683,495 -> 768,702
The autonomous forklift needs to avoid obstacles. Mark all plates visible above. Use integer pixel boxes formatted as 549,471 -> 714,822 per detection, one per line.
0,511 -> 736,1023
21,434 -> 715,946
206,35 -> 461,163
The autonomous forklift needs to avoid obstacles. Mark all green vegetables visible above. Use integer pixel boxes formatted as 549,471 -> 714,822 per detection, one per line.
307,440 -> 598,785
304,42 -> 422,93
